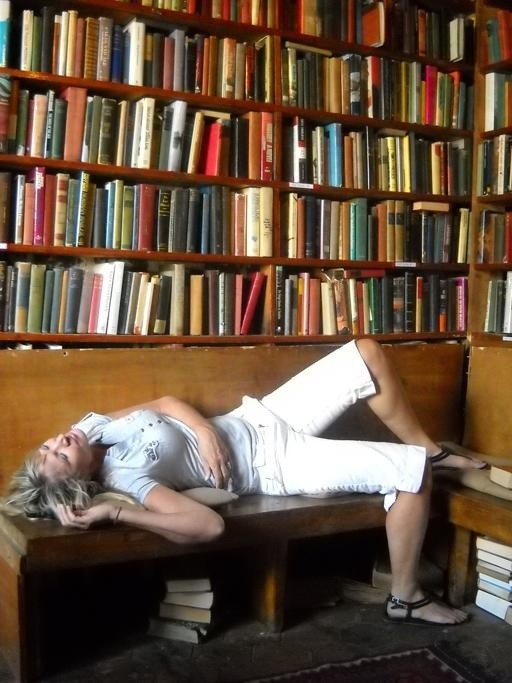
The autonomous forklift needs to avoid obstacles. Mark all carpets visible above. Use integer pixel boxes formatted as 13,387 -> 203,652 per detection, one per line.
238,643 -> 485,682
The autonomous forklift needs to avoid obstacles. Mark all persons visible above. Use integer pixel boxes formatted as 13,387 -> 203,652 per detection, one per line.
3,336 -> 490,627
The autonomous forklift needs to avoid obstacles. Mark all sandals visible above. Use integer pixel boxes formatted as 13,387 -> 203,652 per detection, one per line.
429,446 -> 488,470
382,593 -> 472,628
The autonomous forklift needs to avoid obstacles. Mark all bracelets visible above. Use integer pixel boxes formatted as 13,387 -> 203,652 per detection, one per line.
114,503 -> 126,524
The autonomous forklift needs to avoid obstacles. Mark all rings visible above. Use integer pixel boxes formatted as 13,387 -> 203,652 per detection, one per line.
227,460 -> 232,470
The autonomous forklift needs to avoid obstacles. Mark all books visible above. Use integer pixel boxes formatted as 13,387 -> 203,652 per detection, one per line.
0,0 -> 511,336
157,602 -> 212,625
164,577 -> 212,593
162,593 -> 215,609
145,619 -> 210,645
473,535 -> 512,625
